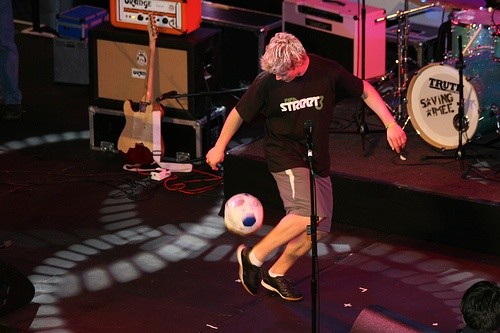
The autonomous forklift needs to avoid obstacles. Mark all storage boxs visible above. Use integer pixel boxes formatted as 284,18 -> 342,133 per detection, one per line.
53,0 -> 226,166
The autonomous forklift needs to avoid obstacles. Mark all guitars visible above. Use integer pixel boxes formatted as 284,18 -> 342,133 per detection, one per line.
119,11 -> 166,160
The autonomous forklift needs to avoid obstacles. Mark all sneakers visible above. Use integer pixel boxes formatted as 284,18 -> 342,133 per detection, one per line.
260,269 -> 304,301
237,244 -> 262,296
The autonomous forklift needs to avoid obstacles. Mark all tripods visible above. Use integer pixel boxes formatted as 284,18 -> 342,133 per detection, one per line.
420,33 -> 500,179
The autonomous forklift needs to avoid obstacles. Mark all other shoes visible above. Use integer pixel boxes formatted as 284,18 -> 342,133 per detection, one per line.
2,103 -> 24,121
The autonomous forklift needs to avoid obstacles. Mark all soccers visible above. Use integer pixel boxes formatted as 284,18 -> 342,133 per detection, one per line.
225,192 -> 264,236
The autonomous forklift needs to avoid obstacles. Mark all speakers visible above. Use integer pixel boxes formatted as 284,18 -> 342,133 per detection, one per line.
350,304 -> 433,333
283,0 -> 387,82
88,20 -> 223,120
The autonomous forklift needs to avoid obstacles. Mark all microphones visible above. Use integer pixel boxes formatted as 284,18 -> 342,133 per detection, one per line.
303,120 -> 314,157
156,91 -> 177,102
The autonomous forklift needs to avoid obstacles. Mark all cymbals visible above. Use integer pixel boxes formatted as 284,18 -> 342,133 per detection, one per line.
408,0 -> 486,9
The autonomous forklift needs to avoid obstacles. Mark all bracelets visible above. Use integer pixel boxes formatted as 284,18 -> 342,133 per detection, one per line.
386,122 -> 397,128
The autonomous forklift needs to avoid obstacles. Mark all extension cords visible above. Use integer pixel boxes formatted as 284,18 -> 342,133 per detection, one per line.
157,162 -> 192,172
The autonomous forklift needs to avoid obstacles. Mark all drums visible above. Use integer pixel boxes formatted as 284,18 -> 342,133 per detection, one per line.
405,49 -> 500,151
450,8 -> 500,59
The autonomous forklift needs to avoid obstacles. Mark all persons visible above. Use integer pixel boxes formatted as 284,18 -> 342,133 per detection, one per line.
456,280 -> 500,333
206,32 -> 407,301
0,0 -> 22,119
